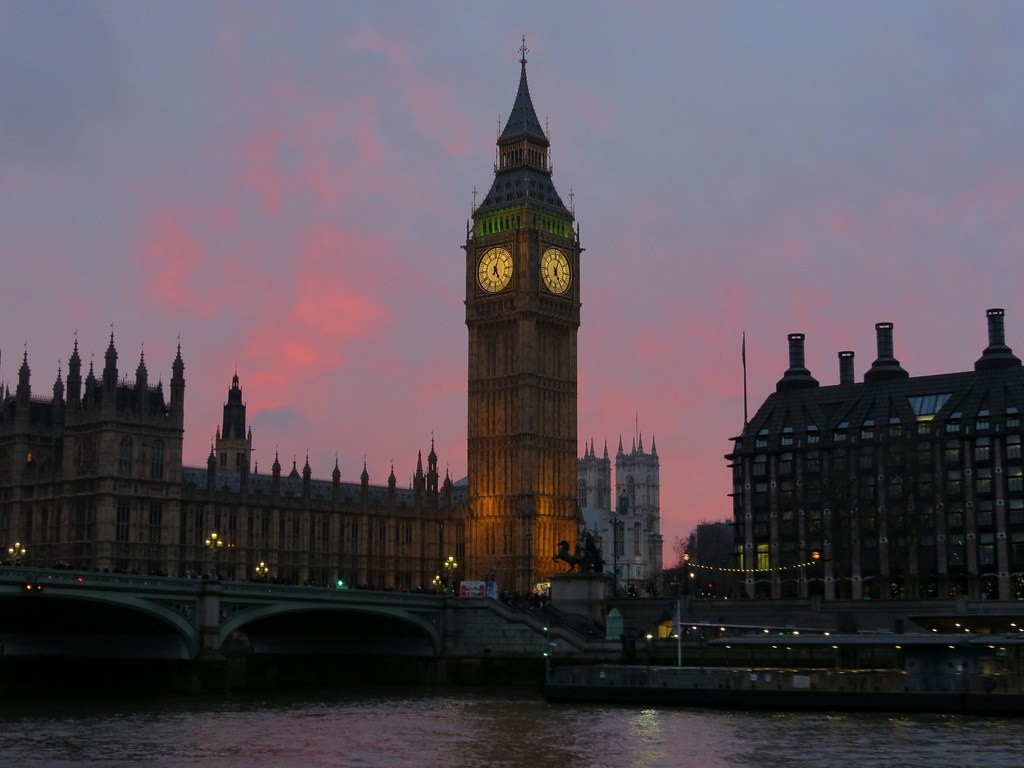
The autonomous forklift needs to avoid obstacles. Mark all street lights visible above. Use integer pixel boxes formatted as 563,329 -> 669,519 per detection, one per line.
683,553 -> 691,589
254,562 -> 269,582
8,542 -> 27,566
204,533 -> 223,578
812,551 -> 822,595
433,575 -> 443,595
444,555 -> 460,594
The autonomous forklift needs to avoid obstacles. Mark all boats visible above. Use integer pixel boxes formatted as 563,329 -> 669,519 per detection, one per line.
542,598 -> 1024,719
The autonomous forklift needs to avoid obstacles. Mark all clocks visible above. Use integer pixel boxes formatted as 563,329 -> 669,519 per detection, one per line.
476,245 -> 514,294
540,247 -> 573,295
